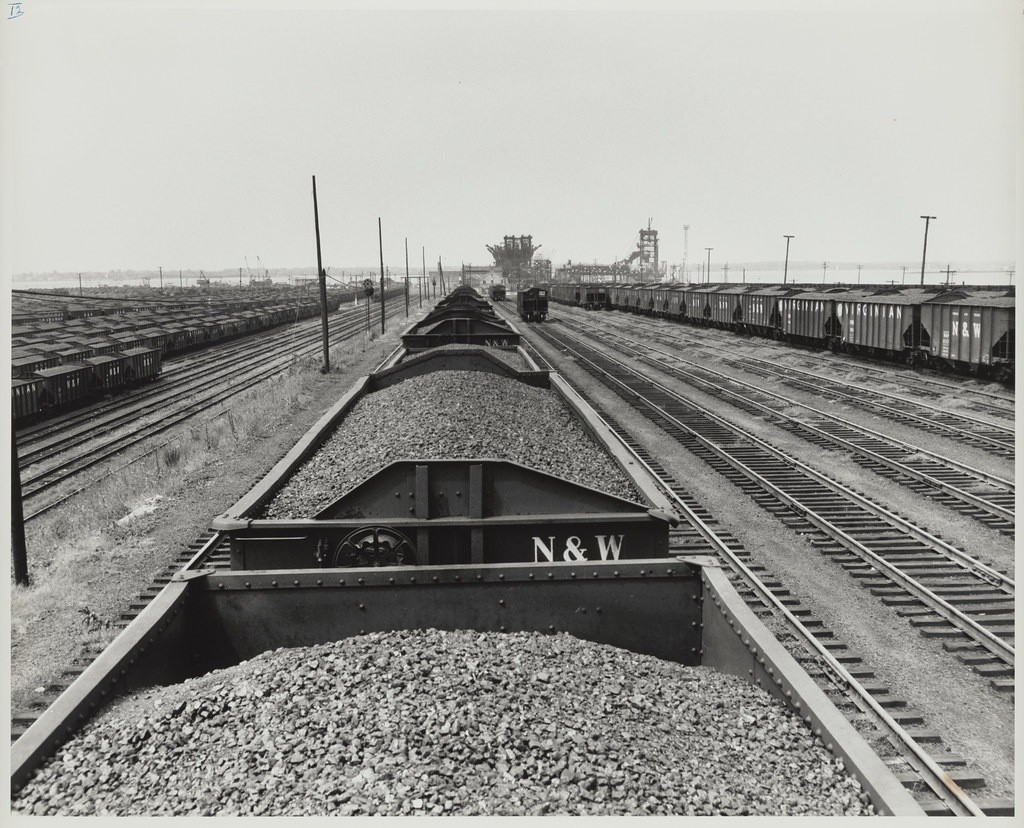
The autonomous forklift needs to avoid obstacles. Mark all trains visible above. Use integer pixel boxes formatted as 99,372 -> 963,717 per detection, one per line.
536,268 -> 1016,391
516,287 -> 549,323
489,284 -> 507,302
12,275 -> 936,817
12,272 -> 380,429
